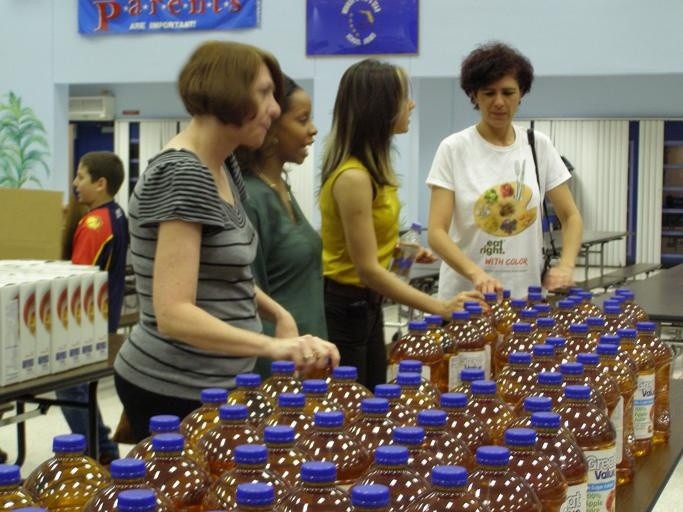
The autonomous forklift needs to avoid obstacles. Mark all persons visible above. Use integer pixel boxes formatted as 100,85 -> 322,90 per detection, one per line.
314,57 -> 492,393
230,74 -> 330,385
112,40 -> 341,452
423,39 -> 583,316
51,149 -> 128,466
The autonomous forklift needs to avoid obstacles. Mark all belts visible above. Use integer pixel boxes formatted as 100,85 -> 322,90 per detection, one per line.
325,281 -> 384,305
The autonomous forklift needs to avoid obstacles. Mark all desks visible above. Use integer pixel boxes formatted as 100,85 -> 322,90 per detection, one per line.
0,337 -> 122,467
382,231 -> 682,512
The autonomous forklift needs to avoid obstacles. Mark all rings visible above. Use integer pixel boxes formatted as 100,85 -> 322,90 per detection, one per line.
302,354 -> 314,363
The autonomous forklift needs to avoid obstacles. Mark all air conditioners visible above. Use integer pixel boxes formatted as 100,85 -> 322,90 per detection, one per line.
69,96 -> 114,121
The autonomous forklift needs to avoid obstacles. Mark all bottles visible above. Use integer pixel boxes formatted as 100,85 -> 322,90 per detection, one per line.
0,282 -> 672,512
390,221 -> 422,283
